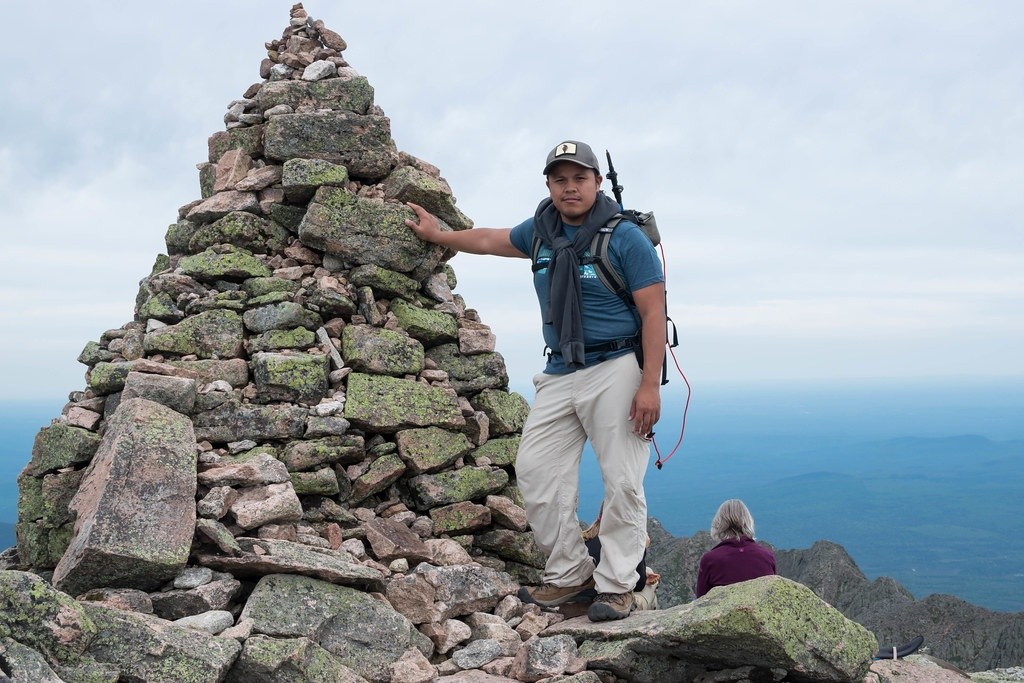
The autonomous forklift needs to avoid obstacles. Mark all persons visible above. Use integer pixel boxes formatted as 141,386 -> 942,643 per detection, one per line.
404,142 -> 667,621
696,498 -> 778,598
584,498 -> 661,611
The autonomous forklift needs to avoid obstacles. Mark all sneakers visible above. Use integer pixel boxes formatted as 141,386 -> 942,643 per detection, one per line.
519,574 -> 596,607
588,592 -> 637,621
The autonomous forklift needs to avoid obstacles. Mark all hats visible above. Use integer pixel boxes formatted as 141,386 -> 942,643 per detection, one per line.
542,141 -> 600,176
